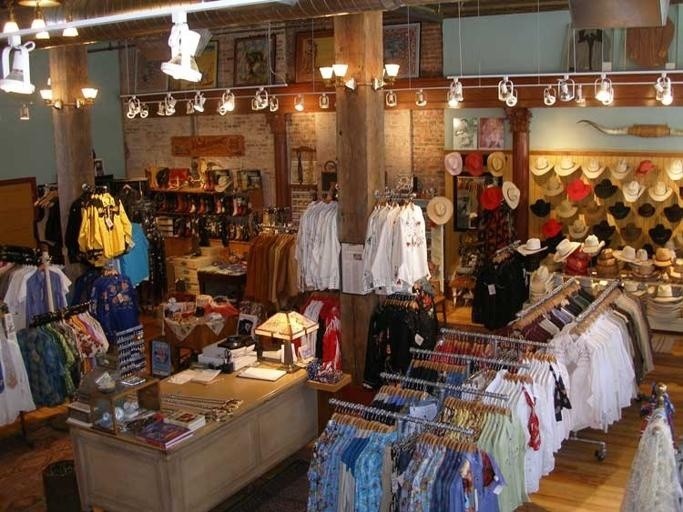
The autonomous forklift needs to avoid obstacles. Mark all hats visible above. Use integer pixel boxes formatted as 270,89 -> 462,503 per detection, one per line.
214,175 -> 233,193
427,197 -> 452,226
515,158 -> 682,305
445,152 -> 521,210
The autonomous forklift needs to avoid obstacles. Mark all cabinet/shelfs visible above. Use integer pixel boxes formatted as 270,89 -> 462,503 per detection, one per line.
151,187 -> 265,239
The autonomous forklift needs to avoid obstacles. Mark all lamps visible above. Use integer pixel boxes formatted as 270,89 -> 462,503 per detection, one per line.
254,310 -> 320,373
0,0 -> 683,120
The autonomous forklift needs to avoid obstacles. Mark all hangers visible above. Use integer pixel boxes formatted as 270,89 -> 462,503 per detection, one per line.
0,185 -> 118,328
267,190 -> 414,240
506,278 -> 628,350
381,277 -> 441,311
311,329 -> 557,450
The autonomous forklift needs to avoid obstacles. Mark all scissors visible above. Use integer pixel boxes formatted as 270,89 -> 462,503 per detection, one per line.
224,350 -> 231,364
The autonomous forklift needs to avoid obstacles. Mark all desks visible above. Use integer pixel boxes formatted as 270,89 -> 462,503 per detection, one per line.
70,358 -> 318,512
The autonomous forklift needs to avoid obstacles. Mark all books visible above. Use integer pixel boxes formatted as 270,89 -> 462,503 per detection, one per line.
135,410 -> 206,450
237,366 -> 287,382
192,369 -> 222,382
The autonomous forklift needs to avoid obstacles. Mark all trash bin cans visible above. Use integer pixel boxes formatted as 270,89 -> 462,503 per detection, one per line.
41,460 -> 81,511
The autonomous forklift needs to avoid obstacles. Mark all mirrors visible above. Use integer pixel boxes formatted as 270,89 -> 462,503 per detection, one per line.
453,172 -> 502,231
